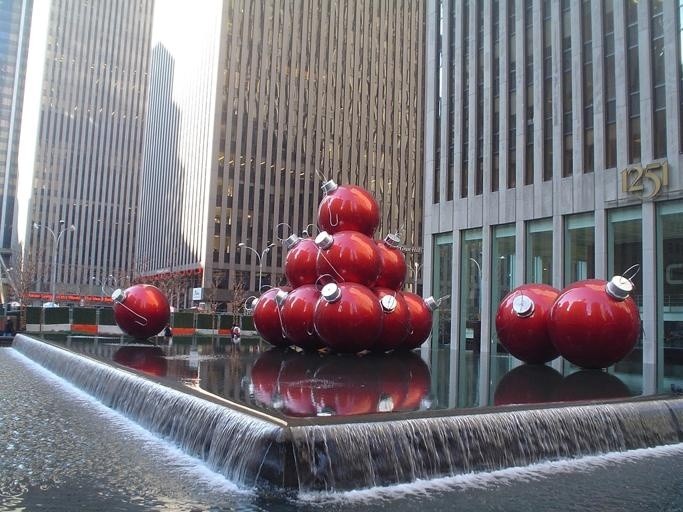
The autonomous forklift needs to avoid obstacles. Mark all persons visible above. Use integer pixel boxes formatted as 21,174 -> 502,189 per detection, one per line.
164,323 -> 172,342
2,315 -> 13,341
230,322 -> 241,343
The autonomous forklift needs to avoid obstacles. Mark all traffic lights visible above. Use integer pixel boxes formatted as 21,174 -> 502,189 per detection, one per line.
408,262 -> 423,294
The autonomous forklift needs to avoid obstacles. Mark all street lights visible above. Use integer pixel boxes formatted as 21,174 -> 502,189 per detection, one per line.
33,220 -> 75,309
239,242 -> 276,292
92,275 -> 130,296
469,252 -> 505,315
